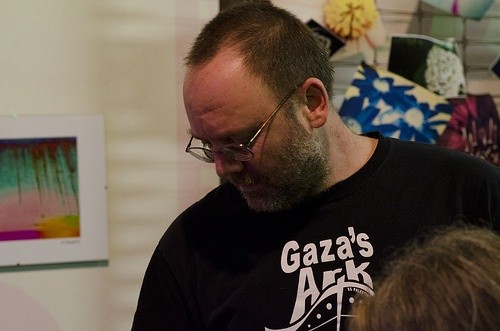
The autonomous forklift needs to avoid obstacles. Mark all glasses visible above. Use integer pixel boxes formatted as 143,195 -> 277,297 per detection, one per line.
186,83 -> 303,164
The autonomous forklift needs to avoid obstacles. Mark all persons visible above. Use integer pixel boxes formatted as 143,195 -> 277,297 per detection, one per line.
345,219 -> 500,331
131,0 -> 500,331
